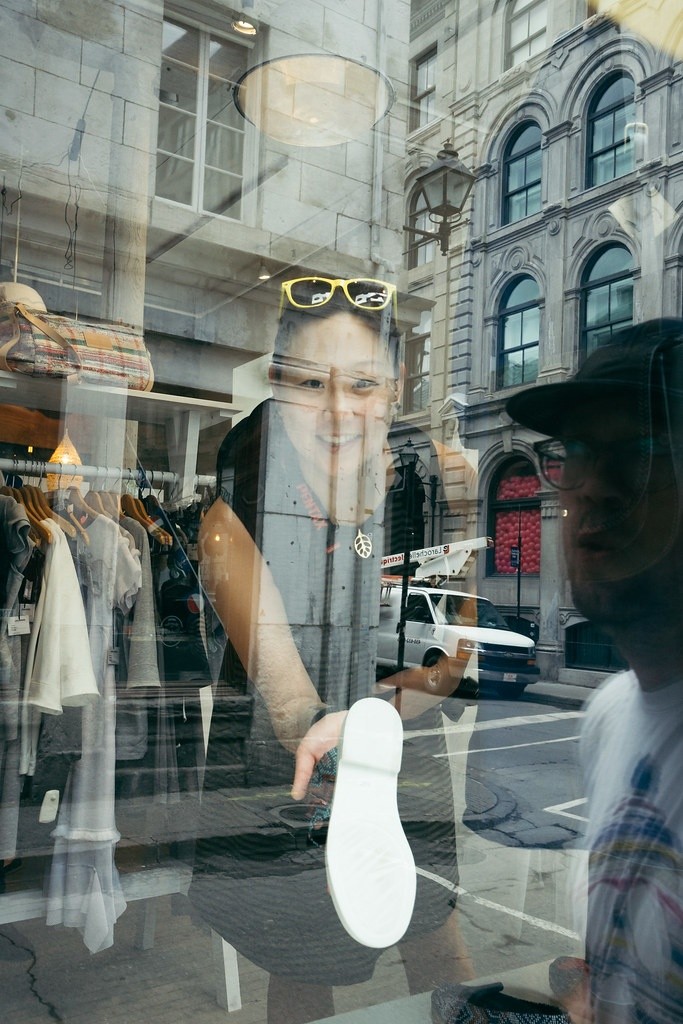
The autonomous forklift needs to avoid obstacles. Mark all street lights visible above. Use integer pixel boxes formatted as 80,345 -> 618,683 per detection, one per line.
423,475 -> 441,547
389,437 -> 420,717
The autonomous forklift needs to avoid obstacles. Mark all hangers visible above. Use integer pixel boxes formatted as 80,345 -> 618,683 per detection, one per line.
0,458 -> 211,549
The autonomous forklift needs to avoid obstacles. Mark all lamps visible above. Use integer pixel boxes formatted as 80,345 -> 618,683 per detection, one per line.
403,137 -> 477,257
47,427 -> 84,492
233,53 -> 395,147
230,0 -> 259,36
0,145 -> 47,313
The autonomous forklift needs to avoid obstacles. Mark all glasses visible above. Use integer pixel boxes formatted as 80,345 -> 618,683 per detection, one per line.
277,276 -> 398,331
532,440 -> 683,492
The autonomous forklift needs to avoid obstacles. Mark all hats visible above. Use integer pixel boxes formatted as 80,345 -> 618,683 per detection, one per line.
505,314 -> 682,439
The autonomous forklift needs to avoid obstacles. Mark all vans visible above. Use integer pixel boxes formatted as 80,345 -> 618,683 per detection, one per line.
376,583 -> 541,700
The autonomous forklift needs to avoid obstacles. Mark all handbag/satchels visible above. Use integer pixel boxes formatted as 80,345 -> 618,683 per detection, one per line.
0,302 -> 155,392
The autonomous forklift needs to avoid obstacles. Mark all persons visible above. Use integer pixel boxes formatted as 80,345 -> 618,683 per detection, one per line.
506,318 -> 682,1024
189,274 -> 478,1024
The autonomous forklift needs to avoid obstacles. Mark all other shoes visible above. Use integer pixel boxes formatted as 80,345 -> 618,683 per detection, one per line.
548,955 -> 601,1019
303,697 -> 418,950
424,981 -> 569,1024
